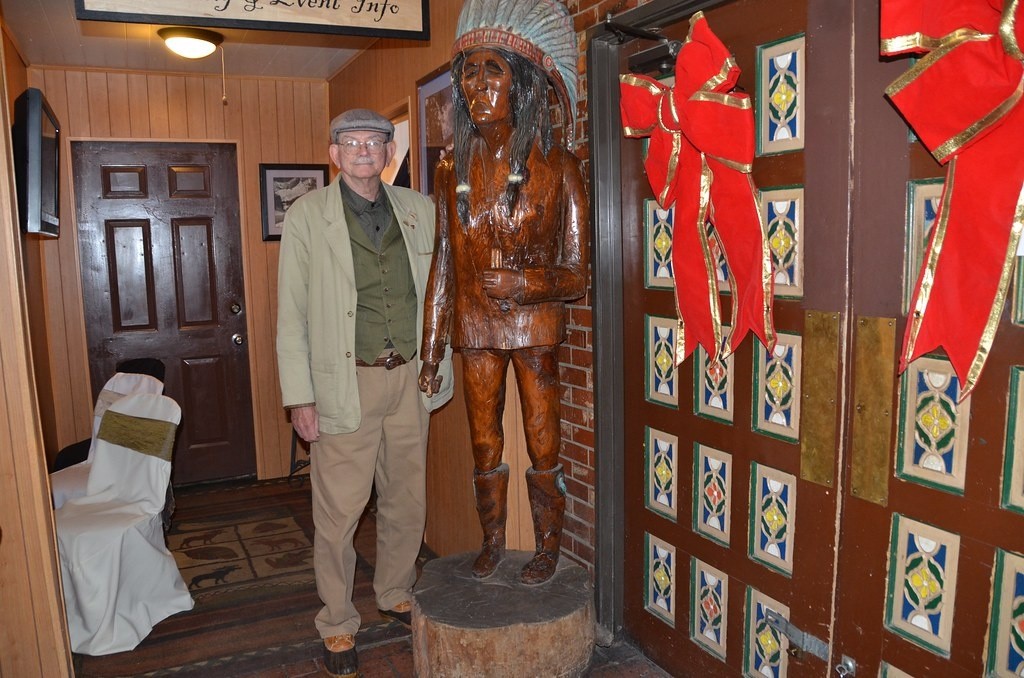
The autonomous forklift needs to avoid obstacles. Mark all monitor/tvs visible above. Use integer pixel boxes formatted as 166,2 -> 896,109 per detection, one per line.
9,86 -> 60,240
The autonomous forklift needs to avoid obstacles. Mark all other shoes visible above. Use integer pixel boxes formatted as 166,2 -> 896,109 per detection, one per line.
391,600 -> 412,612
321,633 -> 360,678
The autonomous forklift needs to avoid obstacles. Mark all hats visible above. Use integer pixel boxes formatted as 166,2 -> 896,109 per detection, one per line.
329,108 -> 395,145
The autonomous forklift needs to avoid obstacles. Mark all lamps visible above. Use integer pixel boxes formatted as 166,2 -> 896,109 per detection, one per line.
157,26 -> 225,61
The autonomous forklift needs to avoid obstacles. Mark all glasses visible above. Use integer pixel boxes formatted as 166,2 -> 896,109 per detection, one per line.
337,140 -> 388,154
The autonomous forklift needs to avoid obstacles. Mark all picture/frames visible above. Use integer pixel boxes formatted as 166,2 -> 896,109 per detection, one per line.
415,62 -> 454,201
259,163 -> 329,242
75,0 -> 431,42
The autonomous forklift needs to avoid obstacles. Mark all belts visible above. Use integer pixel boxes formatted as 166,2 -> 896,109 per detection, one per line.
356,348 -> 417,370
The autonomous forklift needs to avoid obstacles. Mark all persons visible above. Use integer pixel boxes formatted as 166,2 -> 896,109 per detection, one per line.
277,110 -> 455,678
417,0 -> 593,586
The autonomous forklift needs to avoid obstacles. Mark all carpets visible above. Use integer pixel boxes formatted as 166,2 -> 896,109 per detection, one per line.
137,490 -> 375,649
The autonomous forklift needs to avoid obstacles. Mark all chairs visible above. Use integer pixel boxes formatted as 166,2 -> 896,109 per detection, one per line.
50,359 -> 196,657
